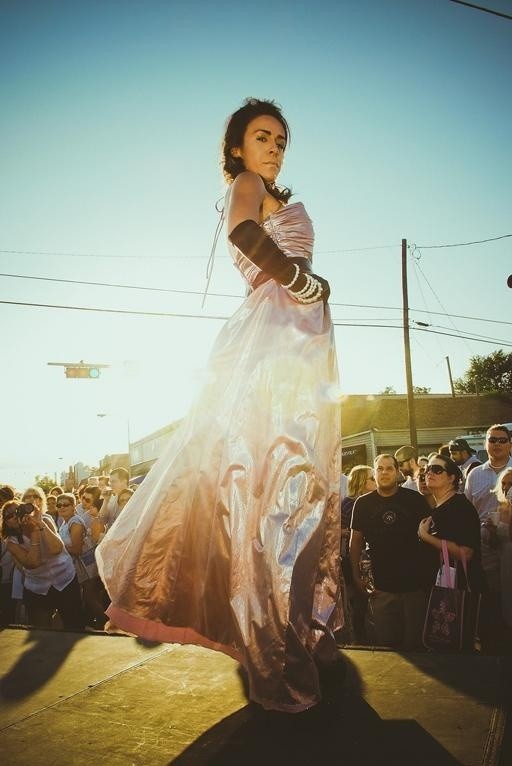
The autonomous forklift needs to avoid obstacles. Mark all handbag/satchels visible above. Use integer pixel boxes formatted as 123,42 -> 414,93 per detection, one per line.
423,542 -> 483,653
66,535 -> 106,585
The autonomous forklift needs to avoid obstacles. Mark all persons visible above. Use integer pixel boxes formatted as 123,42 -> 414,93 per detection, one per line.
394,446 -> 424,496
350,453 -> 432,653
418,452 -> 490,655
75,486 -> 101,524
466,426 -> 512,518
117,488 -> 135,510
439,445 -> 452,459
417,468 -> 436,508
342,466 -> 386,647
481,467 -> 512,655
90,498 -> 103,518
43,496 -> 63,529
0,486 -> 14,625
418,455 -> 428,468
12,486 -> 52,627
448,437 -> 480,492
51,486 -> 63,496
95,99 -> 343,714
98,469 -> 129,532
51,493 -> 106,632
0,502 -> 86,632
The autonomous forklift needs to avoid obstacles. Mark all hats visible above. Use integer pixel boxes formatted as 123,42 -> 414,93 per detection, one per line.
449,438 -> 478,455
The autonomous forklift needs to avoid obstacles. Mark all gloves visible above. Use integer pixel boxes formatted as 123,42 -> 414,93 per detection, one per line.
230,221 -> 331,304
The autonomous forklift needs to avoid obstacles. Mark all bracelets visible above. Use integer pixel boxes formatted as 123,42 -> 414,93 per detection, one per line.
298,282 -> 323,303
295,276 -> 314,297
280,263 -> 300,288
288,273 -> 310,295
293,278 -> 316,298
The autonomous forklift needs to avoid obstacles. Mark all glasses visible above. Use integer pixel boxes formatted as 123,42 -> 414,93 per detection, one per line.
367,475 -> 374,482
397,458 -> 410,467
23,494 -> 38,500
489,437 -> 508,445
81,496 -> 93,506
4,510 -> 19,523
416,473 -> 426,482
55,503 -> 72,508
425,463 -> 451,476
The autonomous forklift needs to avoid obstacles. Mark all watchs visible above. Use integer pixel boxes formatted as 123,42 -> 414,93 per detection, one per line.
40,523 -> 47,531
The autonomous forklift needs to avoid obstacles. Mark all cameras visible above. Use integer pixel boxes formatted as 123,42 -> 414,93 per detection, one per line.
16,503 -> 35,519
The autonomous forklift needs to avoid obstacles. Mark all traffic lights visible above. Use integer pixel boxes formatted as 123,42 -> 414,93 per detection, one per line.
65,366 -> 100,380
